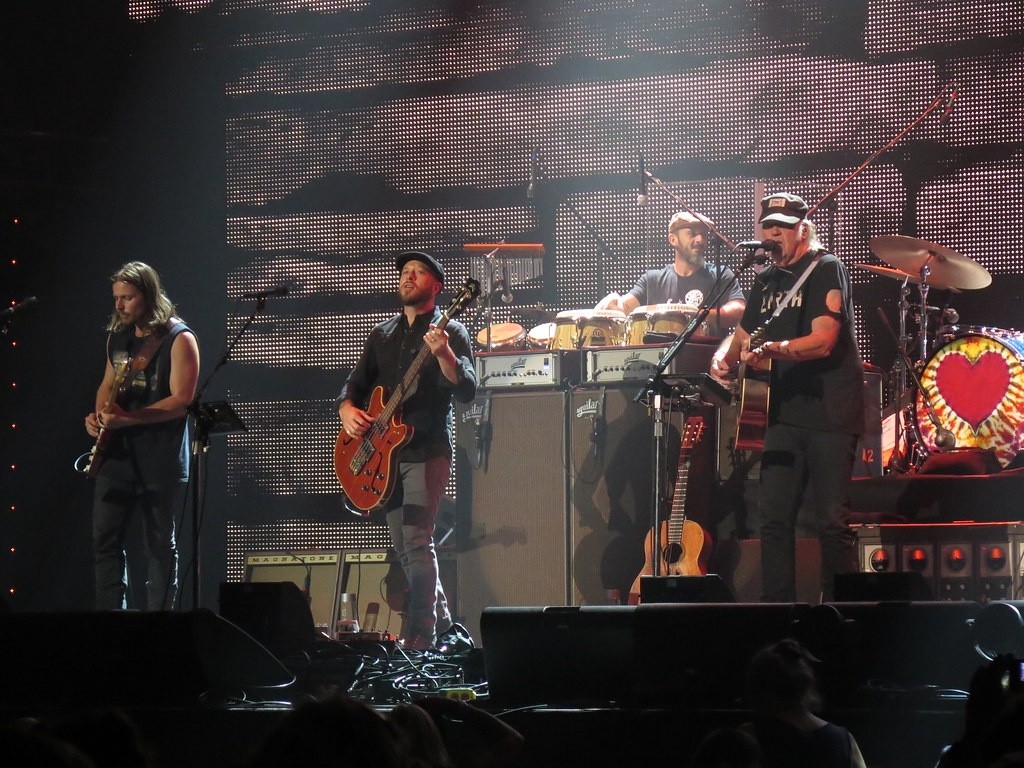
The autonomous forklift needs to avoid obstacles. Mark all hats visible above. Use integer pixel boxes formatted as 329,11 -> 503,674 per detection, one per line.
395,252 -> 444,283
757,192 -> 808,224
669,210 -> 715,233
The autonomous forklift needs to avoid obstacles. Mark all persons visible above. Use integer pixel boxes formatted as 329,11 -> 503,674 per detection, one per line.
245,695 -> 524,768
333,251 -> 477,651
711,193 -> 867,603
686,639 -> 868,768
596,211 -> 747,336
84,261 -> 201,611
936,652 -> 1024,768
0,706 -> 149,768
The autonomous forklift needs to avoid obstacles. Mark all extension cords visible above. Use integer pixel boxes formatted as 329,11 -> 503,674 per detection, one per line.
439,688 -> 477,701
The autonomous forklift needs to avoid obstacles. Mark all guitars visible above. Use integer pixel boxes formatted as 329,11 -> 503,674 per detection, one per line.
626,414 -> 715,607
333,275 -> 483,514
733,324 -> 774,451
84,355 -> 134,484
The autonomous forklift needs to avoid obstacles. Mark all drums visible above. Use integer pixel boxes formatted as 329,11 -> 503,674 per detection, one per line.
552,308 -> 627,348
527,322 -> 557,351
910,330 -> 1023,469
932,325 -> 1020,350
627,303 -> 707,347
476,322 -> 527,351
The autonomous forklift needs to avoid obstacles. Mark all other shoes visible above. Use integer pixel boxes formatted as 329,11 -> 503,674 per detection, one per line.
430,631 -> 460,656
395,647 -> 436,657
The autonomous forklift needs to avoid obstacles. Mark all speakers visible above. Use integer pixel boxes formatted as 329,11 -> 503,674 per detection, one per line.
244,549 -> 342,637
331,550 -> 458,640
456,371 -> 882,650
480,571 -> 1024,707
0,580 -> 317,701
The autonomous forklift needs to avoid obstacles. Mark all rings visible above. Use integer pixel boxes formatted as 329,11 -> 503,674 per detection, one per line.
431,339 -> 436,343
347,431 -> 350,433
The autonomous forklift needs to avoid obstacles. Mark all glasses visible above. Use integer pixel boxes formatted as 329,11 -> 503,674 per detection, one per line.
762,220 -> 794,230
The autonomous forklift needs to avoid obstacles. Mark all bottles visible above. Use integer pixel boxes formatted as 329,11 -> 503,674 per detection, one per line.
361,601 -> 380,632
341,592 -> 358,631
315,622 -> 328,627
382,630 -> 399,641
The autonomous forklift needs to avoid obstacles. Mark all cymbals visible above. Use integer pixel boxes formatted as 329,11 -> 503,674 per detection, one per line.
510,307 -> 553,321
852,260 -> 965,295
870,234 -> 994,290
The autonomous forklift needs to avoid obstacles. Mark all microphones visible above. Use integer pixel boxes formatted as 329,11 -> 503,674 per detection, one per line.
300,574 -> 310,599
913,304 -> 959,323
474,426 -> 482,448
242,285 -> 288,298
527,149 -> 538,198
637,154 -> 648,207
935,435 -> 953,447
501,267 -> 513,303
740,239 -> 778,252
